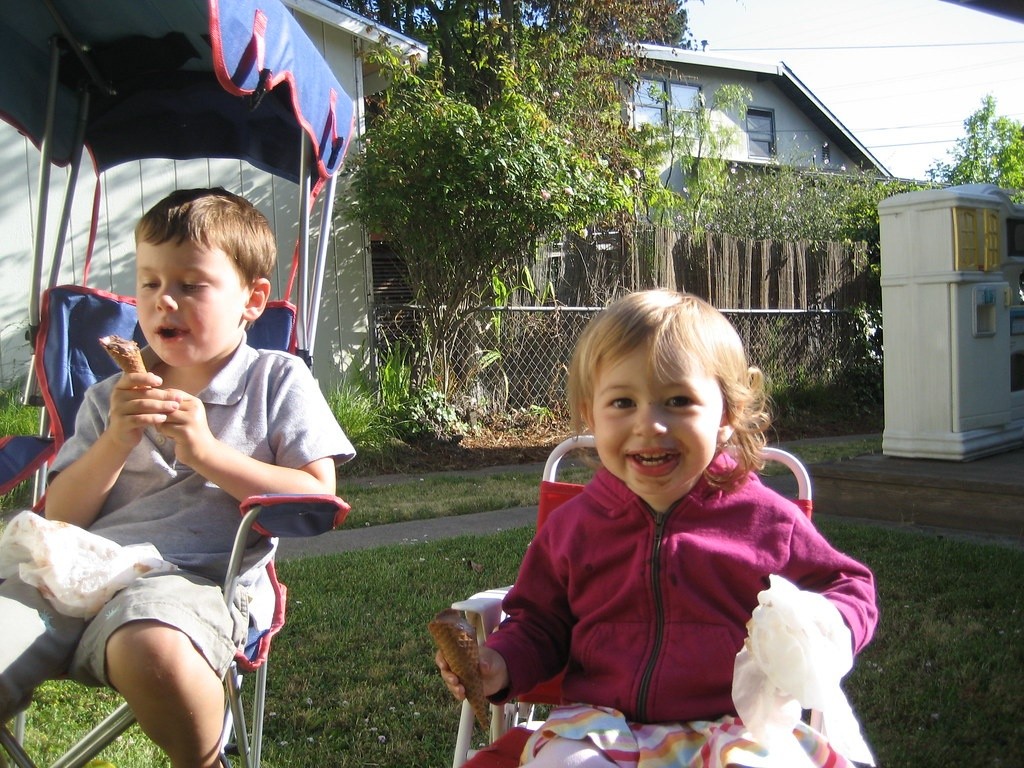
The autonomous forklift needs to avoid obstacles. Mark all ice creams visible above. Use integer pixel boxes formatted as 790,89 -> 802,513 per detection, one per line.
99,334 -> 149,373
429,608 -> 489,730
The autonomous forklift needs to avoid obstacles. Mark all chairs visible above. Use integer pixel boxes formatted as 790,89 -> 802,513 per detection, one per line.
451,436 -> 811,767
0,287 -> 351,768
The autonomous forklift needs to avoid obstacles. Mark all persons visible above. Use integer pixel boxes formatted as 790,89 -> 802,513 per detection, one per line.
437,291 -> 878,768
0,187 -> 356,768
